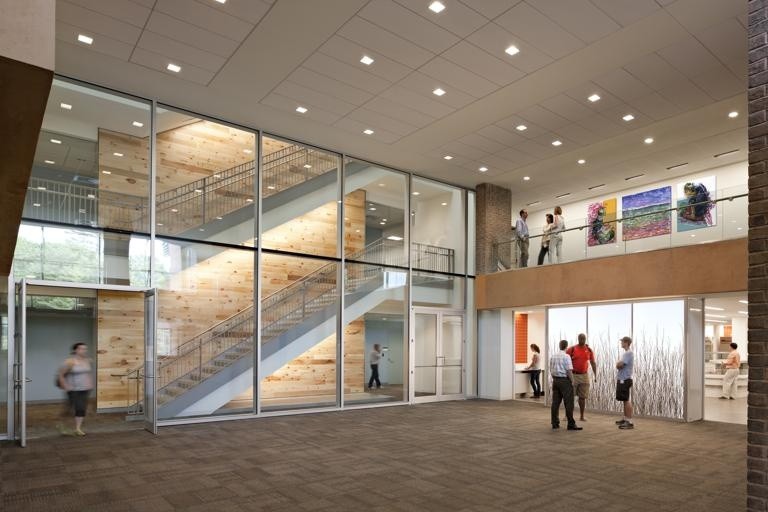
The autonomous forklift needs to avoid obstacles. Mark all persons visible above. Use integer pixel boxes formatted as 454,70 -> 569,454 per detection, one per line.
524,344 -> 541,398
515,209 -> 530,268
543,206 -> 566,265
566,333 -> 597,422
719,343 -> 741,400
549,339 -> 583,429
57,342 -> 94,437
367,344 -> 385,390
614,336 -> 634,430
537,213 -> 554,265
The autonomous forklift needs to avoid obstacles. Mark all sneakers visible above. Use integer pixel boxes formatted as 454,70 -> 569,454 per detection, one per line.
56,424 -> 68,434
616,418 -> 634,429
75,428 -> 87,436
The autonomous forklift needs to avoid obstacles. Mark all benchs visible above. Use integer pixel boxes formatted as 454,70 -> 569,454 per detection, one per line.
704,375 -> 748,398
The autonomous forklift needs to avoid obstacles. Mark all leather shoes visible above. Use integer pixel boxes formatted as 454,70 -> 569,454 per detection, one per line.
552,423 -> 560,430
567,424 -> 584,430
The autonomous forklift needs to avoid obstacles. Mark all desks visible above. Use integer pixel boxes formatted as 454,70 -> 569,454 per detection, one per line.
709,359 -> 748,374
